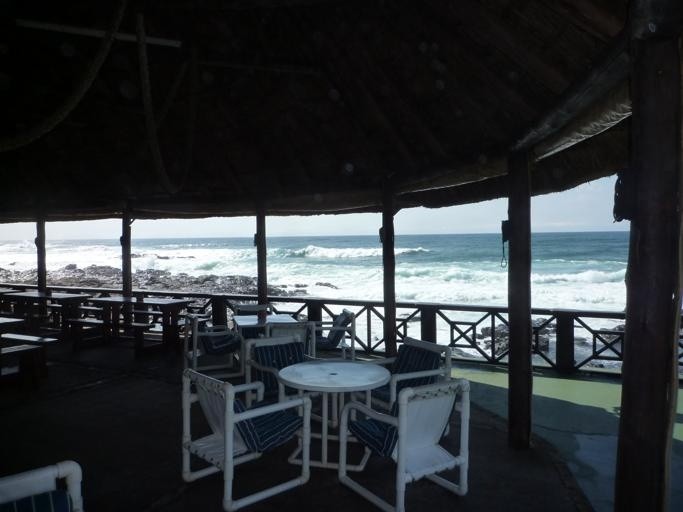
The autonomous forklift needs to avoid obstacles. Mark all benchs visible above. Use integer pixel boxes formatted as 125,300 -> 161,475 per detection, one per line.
1,284 -> 198,384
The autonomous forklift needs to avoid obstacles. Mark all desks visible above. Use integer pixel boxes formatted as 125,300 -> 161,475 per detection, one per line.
279,360 -> 390,471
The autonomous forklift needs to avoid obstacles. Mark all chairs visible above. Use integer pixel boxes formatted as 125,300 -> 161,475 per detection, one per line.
349,337 -> 453,436
243,336 -> 337,431
184,298 -> 355,379
0,460 -> 83,512
339,378 -> 470,512
181,368 -> 312,512
